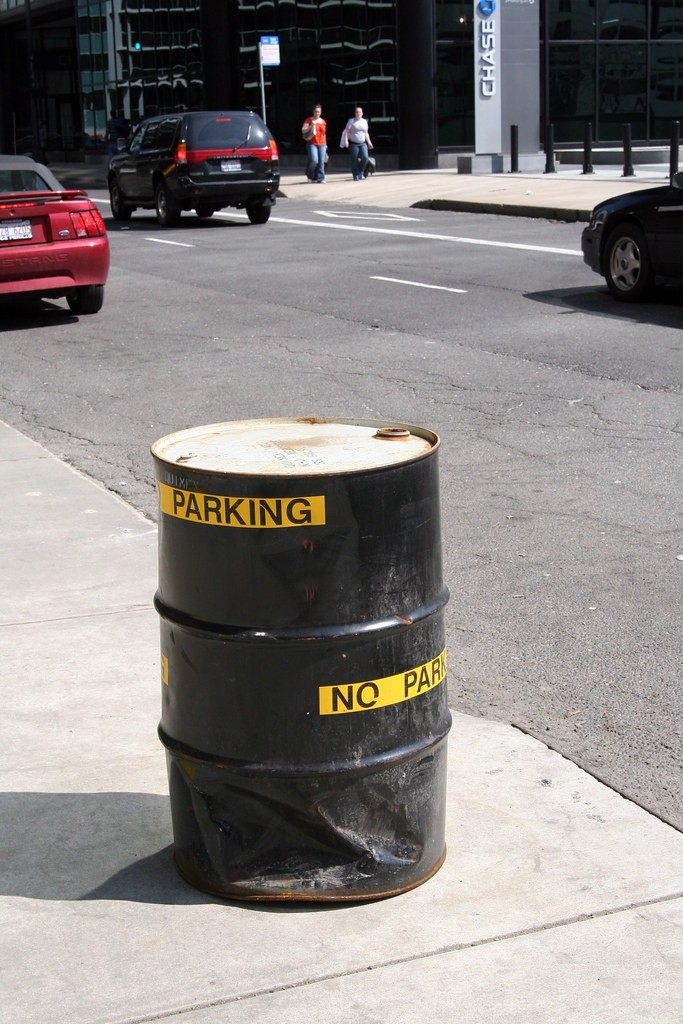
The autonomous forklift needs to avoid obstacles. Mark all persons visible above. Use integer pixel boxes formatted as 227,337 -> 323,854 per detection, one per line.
39,120 -> 49,165
104,110 -> 120,158
346,107 -> 373,181
301,104 -> 327,184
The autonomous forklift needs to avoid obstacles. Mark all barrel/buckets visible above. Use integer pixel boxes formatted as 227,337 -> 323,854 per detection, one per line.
149,415 -> 452,902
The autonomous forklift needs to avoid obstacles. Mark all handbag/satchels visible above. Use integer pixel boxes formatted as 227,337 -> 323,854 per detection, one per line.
127,124 -> 134,140
115,117 -> 122,127
340,119 -> 353,148
302,117 -> 317,141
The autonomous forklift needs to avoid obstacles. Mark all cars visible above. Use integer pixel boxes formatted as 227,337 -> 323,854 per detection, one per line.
0,155 -> 109,315
581,171 -> 683,305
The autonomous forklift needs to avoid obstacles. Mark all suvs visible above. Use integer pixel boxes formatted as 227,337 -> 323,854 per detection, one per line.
107,110 -> 281,229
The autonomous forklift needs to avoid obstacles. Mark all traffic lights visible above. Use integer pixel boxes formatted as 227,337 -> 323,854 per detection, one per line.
135,43 -> 140,50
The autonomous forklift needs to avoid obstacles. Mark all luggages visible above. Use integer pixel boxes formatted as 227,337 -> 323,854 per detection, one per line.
358,147 -> 376,175
305,153 -> 329,180
